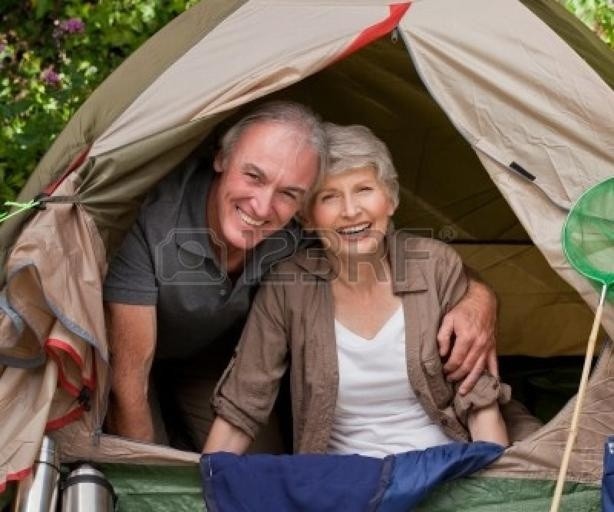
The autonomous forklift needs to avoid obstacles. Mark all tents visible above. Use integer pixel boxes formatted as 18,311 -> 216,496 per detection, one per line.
0,0 -> 614,512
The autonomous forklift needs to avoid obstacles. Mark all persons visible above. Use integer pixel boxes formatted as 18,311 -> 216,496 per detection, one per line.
103,99 -> 329,457
204,123 -> 509,458
